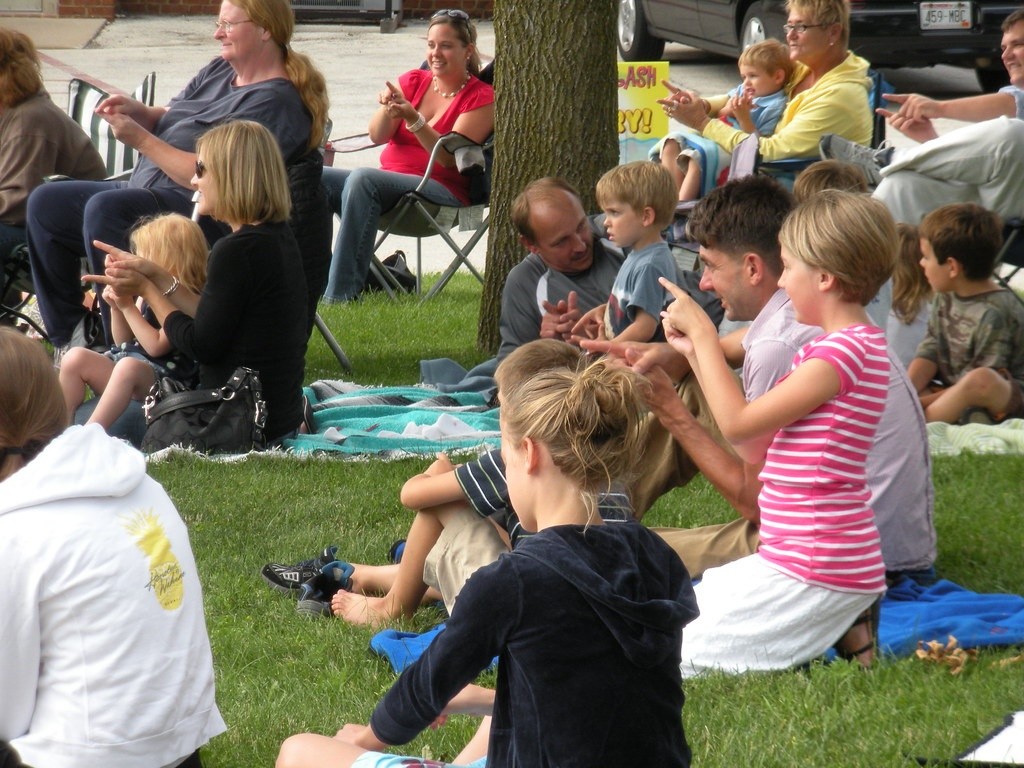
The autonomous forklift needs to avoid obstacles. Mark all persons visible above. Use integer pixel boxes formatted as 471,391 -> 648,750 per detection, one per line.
0,0 -> 1024,768
274,355 -> 697,768
657,190 -> 900,677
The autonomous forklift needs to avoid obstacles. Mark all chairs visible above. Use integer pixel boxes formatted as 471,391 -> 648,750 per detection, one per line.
991,219 -> 1024,307
0,72 -> 159,344
323,57 -> 494,307
758,73 -> 891,188
103,118 -> 354,377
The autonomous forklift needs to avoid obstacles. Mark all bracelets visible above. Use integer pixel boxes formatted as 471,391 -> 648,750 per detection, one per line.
405,111 -> 425,132
163,277 -> 179,297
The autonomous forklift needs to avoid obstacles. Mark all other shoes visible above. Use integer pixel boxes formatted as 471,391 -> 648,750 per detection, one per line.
958,406 -> 998,426
302,395 -> 315,435
388,539 -> 408,563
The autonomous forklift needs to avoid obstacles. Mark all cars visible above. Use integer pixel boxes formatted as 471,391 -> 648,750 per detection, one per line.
616,0 -> 1024,93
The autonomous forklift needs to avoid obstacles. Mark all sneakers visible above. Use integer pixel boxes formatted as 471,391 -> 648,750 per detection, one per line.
296,561 -> 356,617
260,546 -> 337,592
53,310 -> 102,374
818,133 -> 895,192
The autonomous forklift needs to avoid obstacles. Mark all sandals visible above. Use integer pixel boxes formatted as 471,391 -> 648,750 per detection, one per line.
833,602 -> 880,671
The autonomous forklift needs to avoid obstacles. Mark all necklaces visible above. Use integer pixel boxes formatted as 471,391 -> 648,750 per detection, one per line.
433,69 -> 471,99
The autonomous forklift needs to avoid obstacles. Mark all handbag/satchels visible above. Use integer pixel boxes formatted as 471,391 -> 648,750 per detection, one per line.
364,250 -> 416,292
139,367 -> 268,453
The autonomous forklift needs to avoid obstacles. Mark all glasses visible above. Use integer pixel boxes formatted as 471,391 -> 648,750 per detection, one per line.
196,160 -> 205,179
783,23 -> 835,33
431,9 -> 473,42
216,19 -> 253,32
